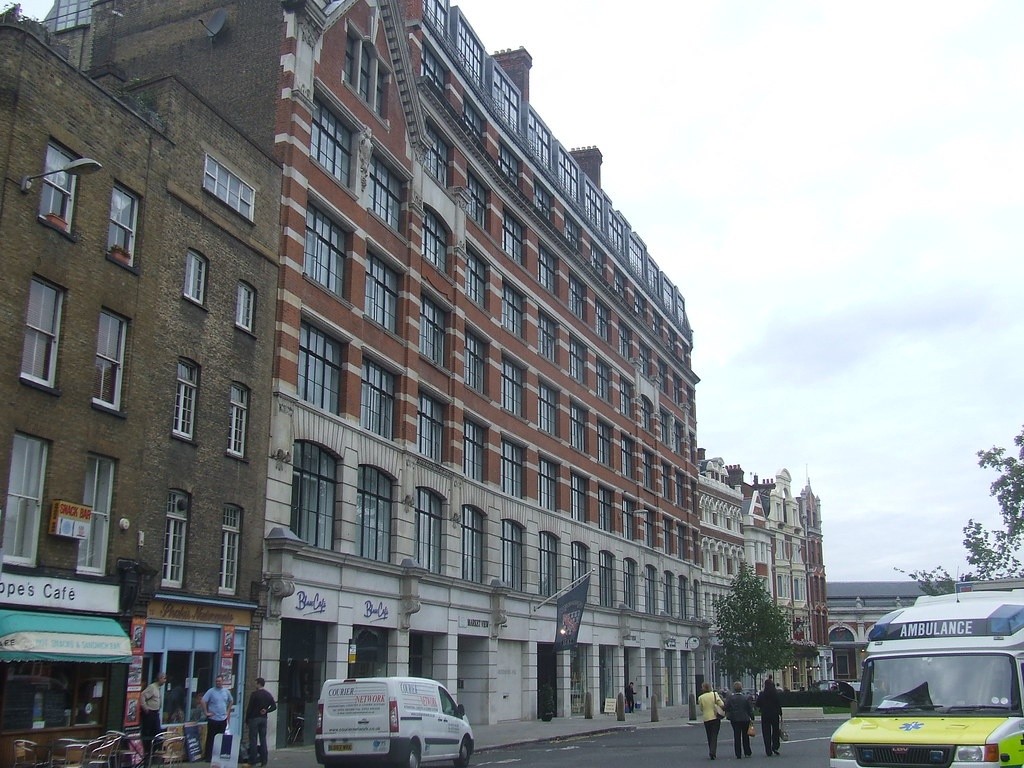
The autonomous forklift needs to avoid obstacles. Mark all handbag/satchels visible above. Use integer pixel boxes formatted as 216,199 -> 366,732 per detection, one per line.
713,692 -> 726,719
779,729 -> 789,741
747,722 -> 756,737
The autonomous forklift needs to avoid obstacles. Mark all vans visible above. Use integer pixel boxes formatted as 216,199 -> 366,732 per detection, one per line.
314,676 -> 475,768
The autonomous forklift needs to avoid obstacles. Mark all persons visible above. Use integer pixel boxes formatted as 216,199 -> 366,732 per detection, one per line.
624,682 -> 637,714
241,677 -> 278,768
139,671 -> 167,767
200,675 -> 234,763
756,679 -> 782,757
725,682 -> 755,759
698,681 -> 726,761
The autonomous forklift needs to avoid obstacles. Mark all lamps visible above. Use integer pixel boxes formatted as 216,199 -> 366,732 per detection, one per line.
20,157 -> 102,195
618,508 -> 649,520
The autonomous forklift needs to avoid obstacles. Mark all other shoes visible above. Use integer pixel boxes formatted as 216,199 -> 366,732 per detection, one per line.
261,762 -> 267,768
773,750 -> 780,756
768,752 -> 773,756
745,751 -> 752,757
632,709 -> 634,712
736,754 -> 741,759
206,757 -> 211,762
709,753 -> 716,760
241,762 -> 256,768
629,710 -> 632,713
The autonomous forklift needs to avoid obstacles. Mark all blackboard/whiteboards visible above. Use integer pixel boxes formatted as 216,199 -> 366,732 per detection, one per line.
183,723 -> 202,762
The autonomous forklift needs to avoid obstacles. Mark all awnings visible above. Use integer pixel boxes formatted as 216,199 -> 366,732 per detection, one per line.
0,608 -> 133,664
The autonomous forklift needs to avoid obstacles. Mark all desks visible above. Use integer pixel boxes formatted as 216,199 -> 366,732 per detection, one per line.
120,733 -> 165,768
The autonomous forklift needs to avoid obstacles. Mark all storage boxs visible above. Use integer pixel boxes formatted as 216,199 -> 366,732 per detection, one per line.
48,499 -> 93,540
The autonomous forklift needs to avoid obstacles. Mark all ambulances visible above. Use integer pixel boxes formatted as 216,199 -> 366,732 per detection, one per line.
828,588 -> 1023,768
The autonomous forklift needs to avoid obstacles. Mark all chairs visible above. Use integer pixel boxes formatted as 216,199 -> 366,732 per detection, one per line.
13,739 -> 52,768
53,730 -> 186,768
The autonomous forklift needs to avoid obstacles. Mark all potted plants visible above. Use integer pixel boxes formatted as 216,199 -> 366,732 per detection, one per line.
541,682 -> 554,721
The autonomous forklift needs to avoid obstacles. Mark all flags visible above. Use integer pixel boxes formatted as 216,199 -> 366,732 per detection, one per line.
550,576 -> 589,655
